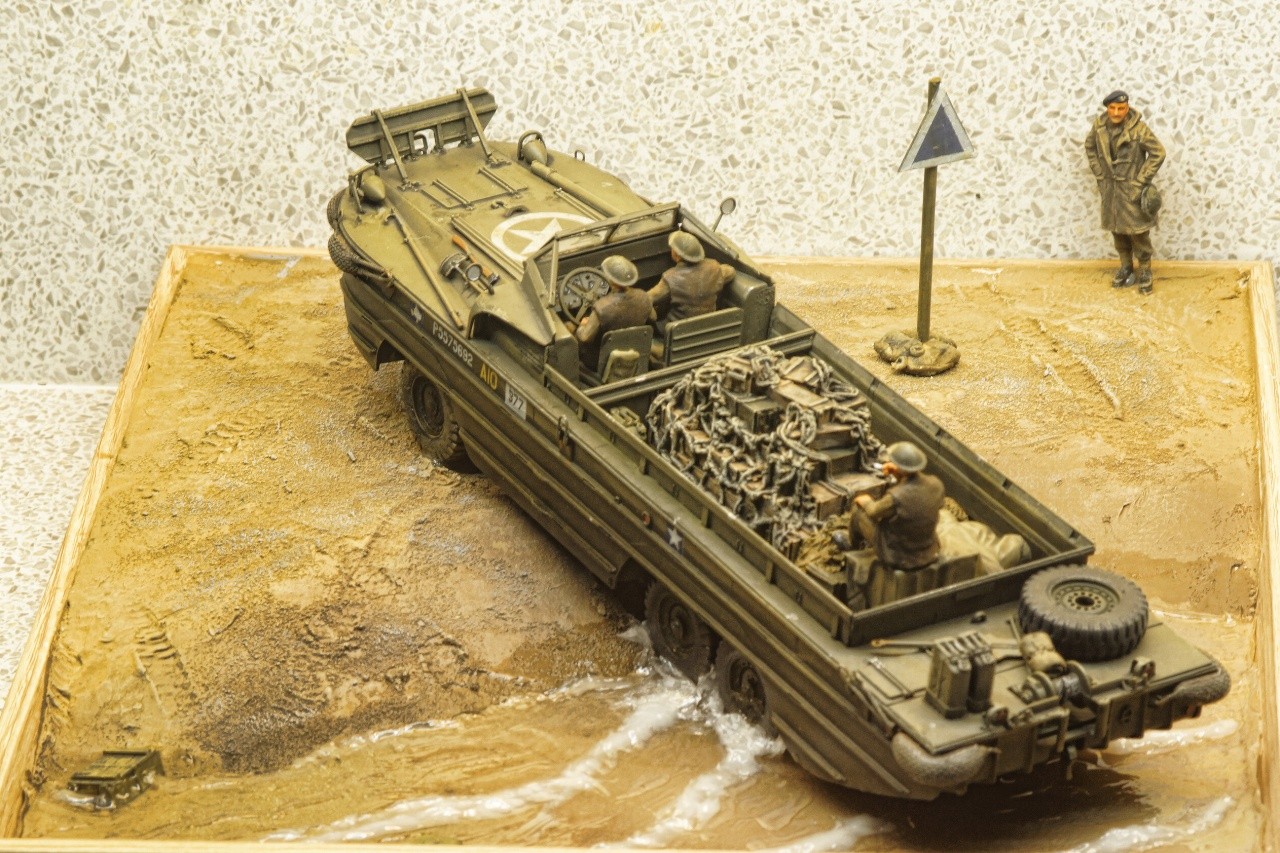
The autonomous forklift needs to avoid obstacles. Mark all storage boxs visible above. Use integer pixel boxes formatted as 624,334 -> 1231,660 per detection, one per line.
68,747 -> 167,810
689,357 -> 890,518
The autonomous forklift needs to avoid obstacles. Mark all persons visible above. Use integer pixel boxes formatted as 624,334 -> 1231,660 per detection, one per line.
832,440 -> 944,570
646,230 -> 734,337
1084,91 -> 1165,293
565,255 -> 658,370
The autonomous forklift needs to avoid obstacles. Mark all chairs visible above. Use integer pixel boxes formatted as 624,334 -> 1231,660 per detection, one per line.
578,326 -> 654,388
650,307 -> 744,369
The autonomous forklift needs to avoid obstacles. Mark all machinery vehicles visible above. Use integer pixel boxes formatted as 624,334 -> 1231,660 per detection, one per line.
325,87 -> 1233,805
